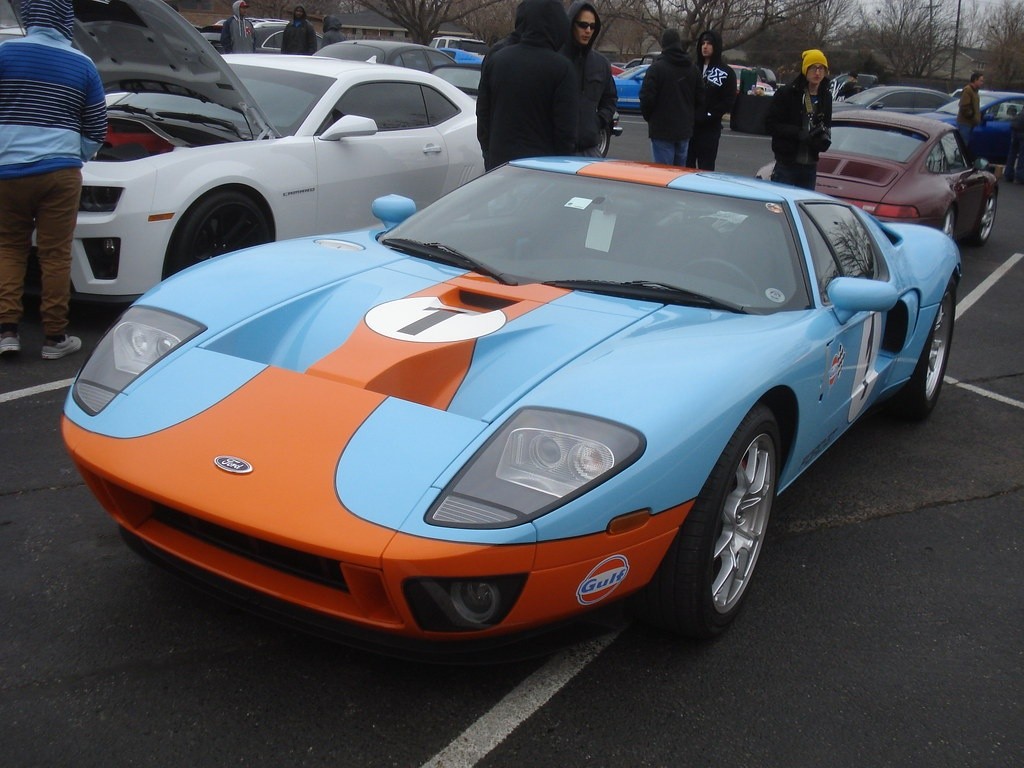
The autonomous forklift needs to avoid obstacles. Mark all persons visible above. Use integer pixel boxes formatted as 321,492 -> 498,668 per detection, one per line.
834,70 -> 864,100
766,46 -> 832,191
740,63 -> 765,92
958,69 -> 984,150
550,0 -> 618,157
640,28 -> 708,167
319,15 -> 345,49
281,5 -> 317,57
221,0 -> 257,54
475,0 -> 581,173
1004,104 -> 1018,121
0,0 -> 109,363
686,29 -> 737,172
1003,105 -> 1024,183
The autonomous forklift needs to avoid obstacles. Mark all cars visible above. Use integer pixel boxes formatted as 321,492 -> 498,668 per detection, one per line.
200,16 -> 489,100
726,65 -> 787,96
1,0 -> 485,298
611,53 -> 662,112
755,73 -> 1024,240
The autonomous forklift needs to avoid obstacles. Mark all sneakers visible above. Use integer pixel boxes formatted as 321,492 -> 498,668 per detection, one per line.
0,332 -> 22,355
41,333 -> 81,360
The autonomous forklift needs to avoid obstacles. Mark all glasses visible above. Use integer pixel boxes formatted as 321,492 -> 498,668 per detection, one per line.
573,19 -> 597,29
808,64 -> 826,72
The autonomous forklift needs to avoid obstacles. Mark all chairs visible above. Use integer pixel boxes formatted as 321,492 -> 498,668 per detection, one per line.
1006,105 -> 1017,116
717,215 -> 797,301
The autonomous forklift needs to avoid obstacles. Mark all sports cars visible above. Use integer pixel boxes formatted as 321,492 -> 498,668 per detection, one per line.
60,157 -> 963,670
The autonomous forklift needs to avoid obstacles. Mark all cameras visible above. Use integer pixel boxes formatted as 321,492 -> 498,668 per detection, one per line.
810,125 -> 831,150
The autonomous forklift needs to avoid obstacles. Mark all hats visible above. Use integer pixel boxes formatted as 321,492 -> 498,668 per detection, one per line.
239,1 -> 250,8
848,70 -> 858,79
661,28 -> 683,51
802,49 -> 828,75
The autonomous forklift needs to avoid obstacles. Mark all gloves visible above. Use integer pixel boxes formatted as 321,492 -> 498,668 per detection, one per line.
801,131 -> 832,153
